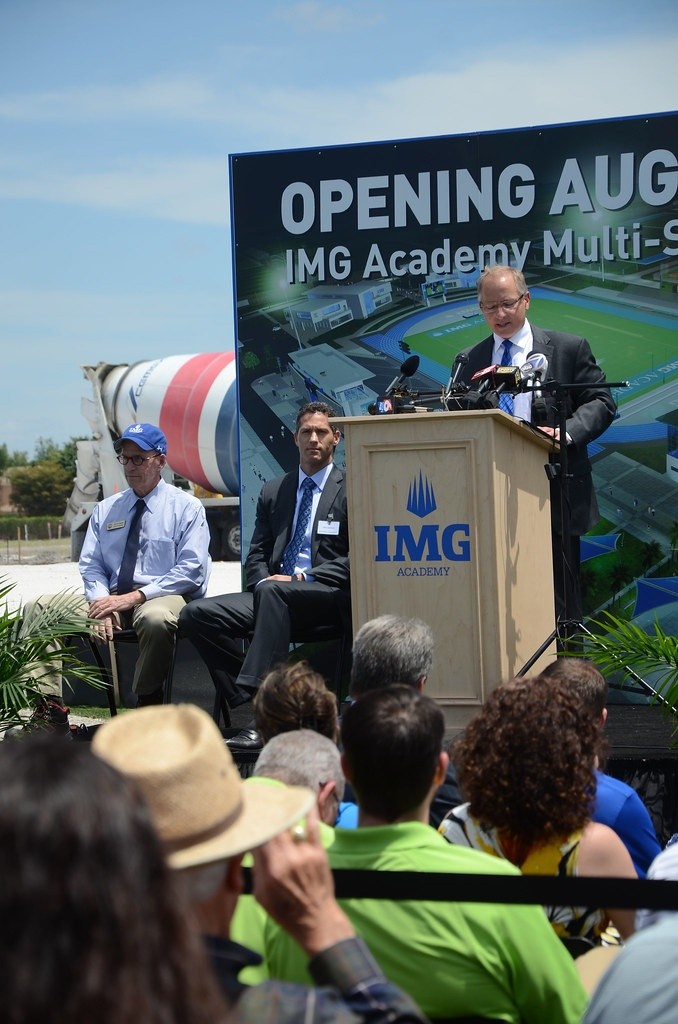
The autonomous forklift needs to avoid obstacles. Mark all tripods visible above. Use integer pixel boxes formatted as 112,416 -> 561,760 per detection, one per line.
408,381 -> 678,718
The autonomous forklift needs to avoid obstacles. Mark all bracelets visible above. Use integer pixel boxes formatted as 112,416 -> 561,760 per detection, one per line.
294,574 -> 299,581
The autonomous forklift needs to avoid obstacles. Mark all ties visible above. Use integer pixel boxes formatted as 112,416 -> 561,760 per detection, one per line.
497,340 -> 514,417
116,499 -> 146,630
280,476 -> 316,576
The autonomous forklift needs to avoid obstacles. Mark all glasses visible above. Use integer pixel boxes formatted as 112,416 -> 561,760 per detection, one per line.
480,293 -> 524,314
116,451 -> 161,466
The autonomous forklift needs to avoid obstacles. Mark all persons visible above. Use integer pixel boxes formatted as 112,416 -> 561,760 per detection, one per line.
448,264 -> 616,660
17,423 -> 213,740
179,402 -> 351,754
0,610 -> 677,1023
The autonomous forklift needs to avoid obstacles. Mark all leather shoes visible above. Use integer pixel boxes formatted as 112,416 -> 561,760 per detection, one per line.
225,714 -> 287,752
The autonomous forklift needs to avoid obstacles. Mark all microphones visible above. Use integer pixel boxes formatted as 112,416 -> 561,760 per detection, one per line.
368,351 -> 549,416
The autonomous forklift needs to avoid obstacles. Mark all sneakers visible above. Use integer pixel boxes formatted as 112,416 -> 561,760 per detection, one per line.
4,700 -> 72,739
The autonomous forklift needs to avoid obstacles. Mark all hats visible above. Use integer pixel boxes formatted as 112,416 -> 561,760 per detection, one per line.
113,422 -> 167,455
90,703 -> 316,869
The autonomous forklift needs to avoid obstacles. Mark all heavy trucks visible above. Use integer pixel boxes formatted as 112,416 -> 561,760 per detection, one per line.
62,348 -> 242,565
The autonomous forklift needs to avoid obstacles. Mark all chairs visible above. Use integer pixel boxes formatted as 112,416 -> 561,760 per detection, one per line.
209,620 -> 347,729
62,552 -> 233,729
